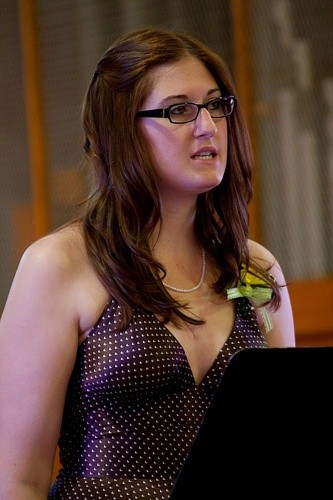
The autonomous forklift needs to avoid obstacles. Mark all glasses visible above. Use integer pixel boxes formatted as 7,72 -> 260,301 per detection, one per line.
136,97 -> 237,124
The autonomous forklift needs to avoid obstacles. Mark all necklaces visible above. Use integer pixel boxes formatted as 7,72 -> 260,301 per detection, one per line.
145,243 -> 206,292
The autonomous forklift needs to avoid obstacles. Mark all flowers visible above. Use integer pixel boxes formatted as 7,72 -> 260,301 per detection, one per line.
226,263 -> 274,333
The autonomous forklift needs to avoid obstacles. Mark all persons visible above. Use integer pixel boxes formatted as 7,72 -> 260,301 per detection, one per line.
1,27 -> 297,498
